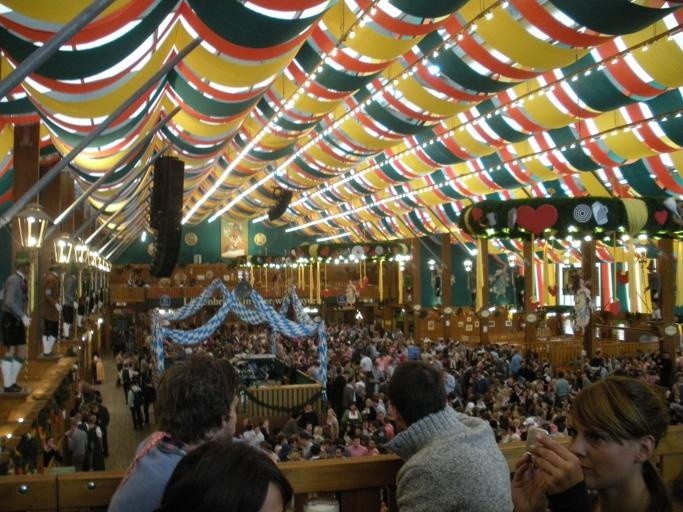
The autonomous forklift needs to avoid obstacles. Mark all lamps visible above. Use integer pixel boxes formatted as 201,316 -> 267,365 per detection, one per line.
12,201 -> 117,278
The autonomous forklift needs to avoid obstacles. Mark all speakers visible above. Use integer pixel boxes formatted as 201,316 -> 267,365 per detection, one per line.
150,156 -> 184,226
149,218 -> 181,277
268,190 -> 292,220
